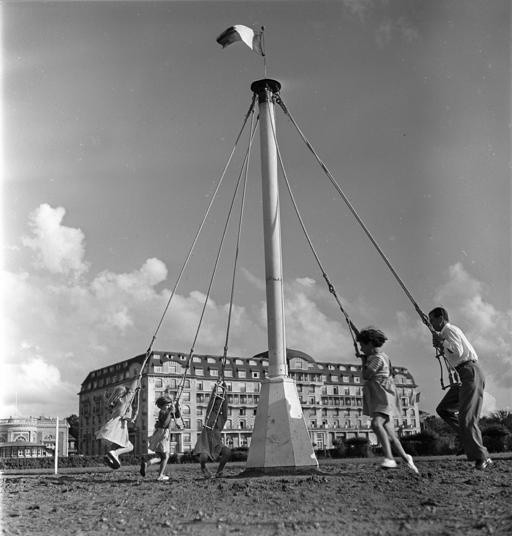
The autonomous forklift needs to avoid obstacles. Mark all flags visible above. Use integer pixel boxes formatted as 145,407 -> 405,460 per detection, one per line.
215,24 -> 265,57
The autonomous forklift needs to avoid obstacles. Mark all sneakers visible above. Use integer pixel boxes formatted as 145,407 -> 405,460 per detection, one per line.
381,458 -> 397,467
103,450 -> 120,469
404,454 -> 419,473
215,472 -> 228,477
156,475 -> 170,481
140,462 -> 146,477
196,472 -> 211,480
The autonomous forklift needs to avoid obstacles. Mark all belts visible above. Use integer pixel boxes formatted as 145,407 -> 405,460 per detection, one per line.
454,360 -> 475,370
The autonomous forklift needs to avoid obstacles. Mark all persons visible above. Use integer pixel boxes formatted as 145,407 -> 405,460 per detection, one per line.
428,307 -> 494,471
192,383 -> 231,478
93,377 -> 141,469
140,397 -> 182,483
356,329 -> 420,476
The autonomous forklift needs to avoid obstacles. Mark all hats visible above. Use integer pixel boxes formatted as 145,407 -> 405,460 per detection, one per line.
156,396 -> 172,408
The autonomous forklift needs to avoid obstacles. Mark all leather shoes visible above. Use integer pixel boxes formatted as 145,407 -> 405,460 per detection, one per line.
476,457 -> 492,470
